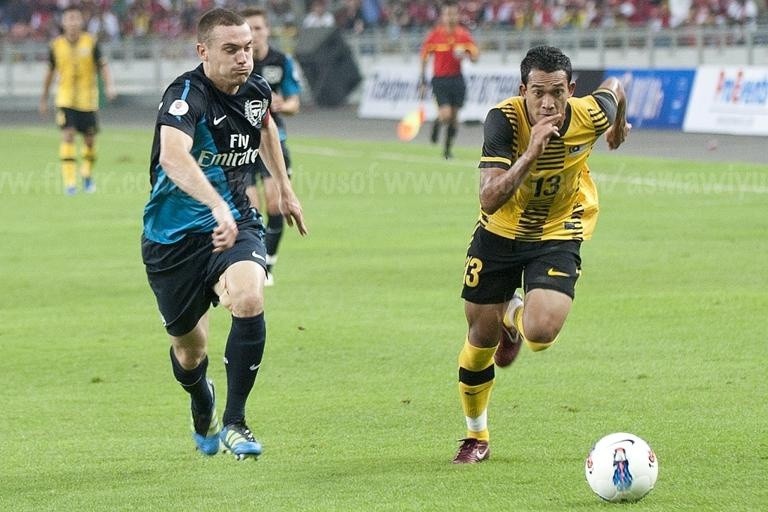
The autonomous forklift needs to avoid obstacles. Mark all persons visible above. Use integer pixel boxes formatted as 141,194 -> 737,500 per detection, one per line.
141,8 -> 306,461
39,6 -> 115,195
460,1 -> 767,32
418,2 -> 479,159
453,47 -> 631,464
238,10 -> 302,286
1,1 -> 439,27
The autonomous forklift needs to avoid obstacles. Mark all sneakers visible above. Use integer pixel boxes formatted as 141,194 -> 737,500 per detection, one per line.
61,176 -> 95,195
450,436 -> 492,466
187,378 -> 222,460
494,294 -> 527,373
218,424 -> 262,463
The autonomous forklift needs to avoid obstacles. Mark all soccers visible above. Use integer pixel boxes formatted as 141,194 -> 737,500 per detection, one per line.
586,432 -> 658,503
